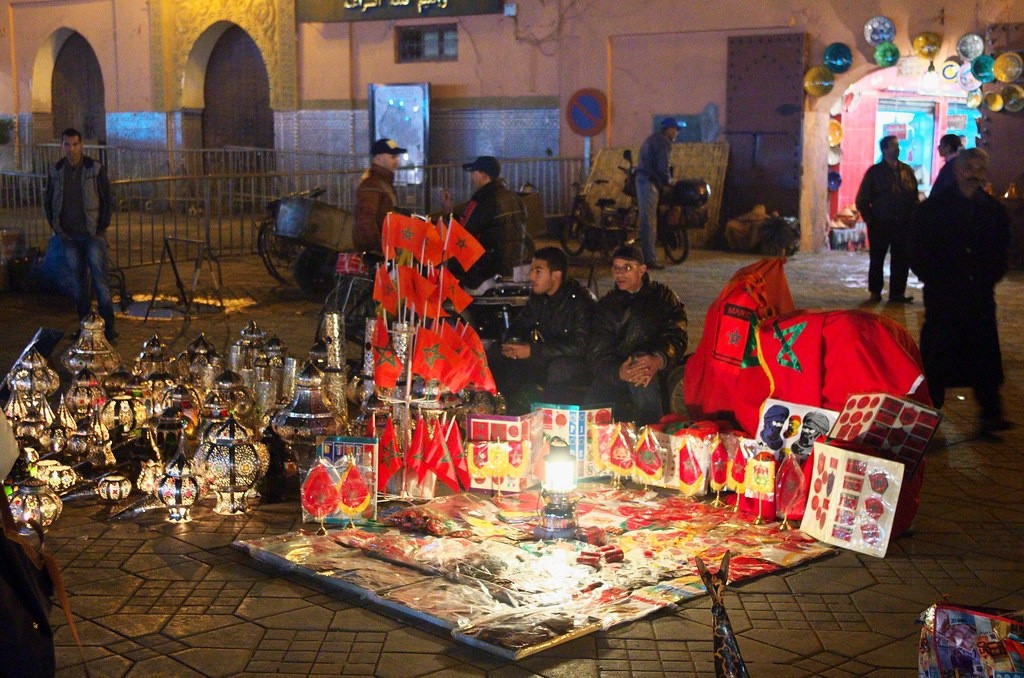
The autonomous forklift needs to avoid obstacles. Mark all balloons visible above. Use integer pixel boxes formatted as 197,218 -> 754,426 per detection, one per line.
827,96 -> 843,192
804,42 -> 853,97
864,15 -> 900,68
914,32 -> 942,61
939,34 -> 1024,112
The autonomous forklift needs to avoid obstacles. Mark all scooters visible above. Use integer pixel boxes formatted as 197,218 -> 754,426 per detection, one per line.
557,178 -> 640,265
616,150 -> 711,265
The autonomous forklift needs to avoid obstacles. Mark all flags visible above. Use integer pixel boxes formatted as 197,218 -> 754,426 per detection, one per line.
373,210 -> 498,395
365,411 -> 472,494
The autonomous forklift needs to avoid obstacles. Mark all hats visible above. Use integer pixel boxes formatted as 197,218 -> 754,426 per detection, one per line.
662,117 -> 683,130
371,139 -> 407,155
613,245 -> 644,266
462,156 -> 501,178
941,134 -> 963,147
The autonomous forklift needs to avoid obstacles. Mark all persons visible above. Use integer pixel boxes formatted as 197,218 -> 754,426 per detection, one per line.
353,138 -> 408,305
906,149 -> 1018,430
929,134 -> 971,202
43,128 -> 119,343
488,246 -> 600,415
0,404 -> 57,677
855,138 -> 920,307
957,133 -> 970,150
587,243 -> 689,427
635,117 -> 684,270
438,155 -> 529,311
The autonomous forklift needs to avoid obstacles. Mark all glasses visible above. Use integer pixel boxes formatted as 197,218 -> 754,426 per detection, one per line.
952,162 -> 987,174
611,265 -> 641,273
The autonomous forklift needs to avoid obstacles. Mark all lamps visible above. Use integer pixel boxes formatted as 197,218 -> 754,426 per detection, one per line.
537,436 -> 580,539
923,60 -> 939,90
3,305 -> 506,535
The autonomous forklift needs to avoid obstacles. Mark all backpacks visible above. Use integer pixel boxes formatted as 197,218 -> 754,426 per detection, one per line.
494,185 -> 549,276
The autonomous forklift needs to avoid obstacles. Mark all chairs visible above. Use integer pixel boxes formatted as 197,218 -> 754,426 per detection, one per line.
0,227 -> 48,300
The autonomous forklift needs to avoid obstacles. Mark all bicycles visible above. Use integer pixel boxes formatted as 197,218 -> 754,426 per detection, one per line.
256,187 -> 327,283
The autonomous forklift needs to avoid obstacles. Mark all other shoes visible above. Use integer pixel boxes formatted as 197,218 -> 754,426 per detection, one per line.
71,326 -> 120,340
871,291 -> 914,302
980,417 -> 1017,431
646,263 -> 665,269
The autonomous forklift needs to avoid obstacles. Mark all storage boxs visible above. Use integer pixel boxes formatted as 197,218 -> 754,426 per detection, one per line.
629,417 -> 730,496
827,389 -> 944,481
670,181 -> 712,207
